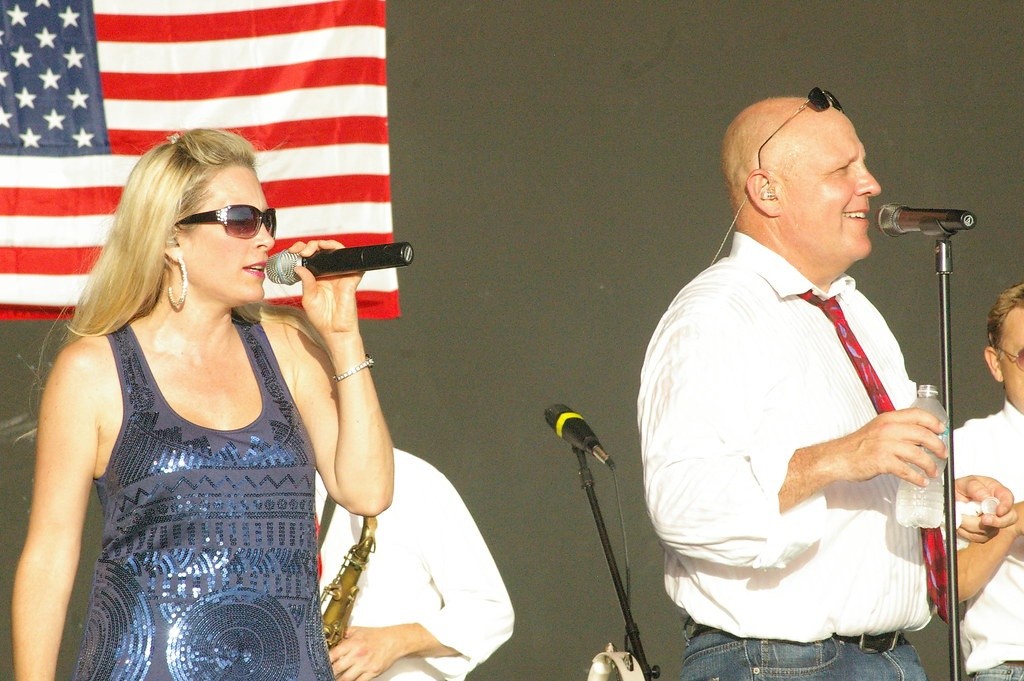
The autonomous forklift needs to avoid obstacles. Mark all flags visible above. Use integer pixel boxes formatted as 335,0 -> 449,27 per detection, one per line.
0,0 -> 402,321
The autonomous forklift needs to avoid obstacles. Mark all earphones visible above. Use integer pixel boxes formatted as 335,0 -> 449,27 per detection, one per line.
761,186 -> 777,200
166,237 -> 179,248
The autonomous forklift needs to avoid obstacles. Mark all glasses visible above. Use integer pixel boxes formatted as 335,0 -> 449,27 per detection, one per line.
995,346 -> 1024,371
174,204 -> 277,239
757,87 -> 843,169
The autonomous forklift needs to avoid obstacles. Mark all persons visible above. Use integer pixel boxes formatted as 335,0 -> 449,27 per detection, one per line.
934,282 -> 1024,681
10,128 -> 394,681
314,448 -> 514,681
634,86 -> 1017,681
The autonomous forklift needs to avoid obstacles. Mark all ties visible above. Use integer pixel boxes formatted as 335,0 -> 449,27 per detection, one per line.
800,291 -> 940,624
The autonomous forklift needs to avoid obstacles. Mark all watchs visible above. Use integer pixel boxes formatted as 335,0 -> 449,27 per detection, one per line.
334,353 -> 375,383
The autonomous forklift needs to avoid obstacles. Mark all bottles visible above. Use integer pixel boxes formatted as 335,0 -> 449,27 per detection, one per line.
895,384 -> 949,530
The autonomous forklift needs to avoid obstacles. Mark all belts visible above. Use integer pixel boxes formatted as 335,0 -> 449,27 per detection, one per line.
684,618 -> 908,651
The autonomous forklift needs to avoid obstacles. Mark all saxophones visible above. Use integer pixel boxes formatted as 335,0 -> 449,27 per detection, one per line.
314,474 -> 385,650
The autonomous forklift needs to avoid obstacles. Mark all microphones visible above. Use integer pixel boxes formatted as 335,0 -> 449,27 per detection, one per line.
545,404 -> 615,470
878,203 -> 977,238
266,241 -> 414,286
